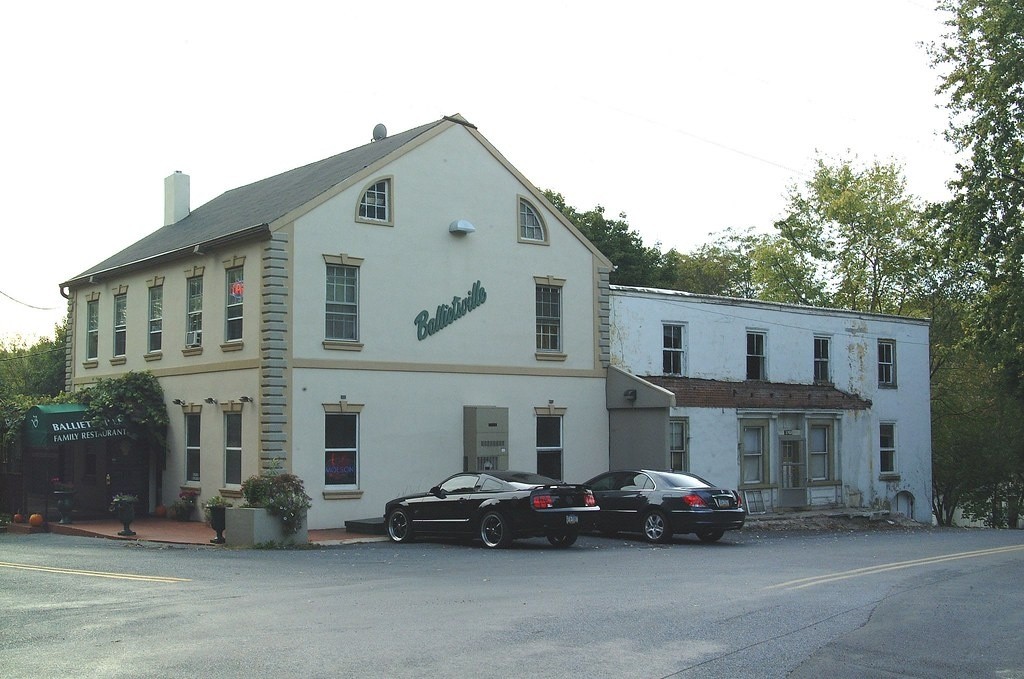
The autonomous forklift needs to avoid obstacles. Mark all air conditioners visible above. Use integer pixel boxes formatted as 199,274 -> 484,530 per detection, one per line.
185,331 -> 200,346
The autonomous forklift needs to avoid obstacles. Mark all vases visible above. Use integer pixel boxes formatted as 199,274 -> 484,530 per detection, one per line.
179,504 -> 194,522
54,492 -> 77,524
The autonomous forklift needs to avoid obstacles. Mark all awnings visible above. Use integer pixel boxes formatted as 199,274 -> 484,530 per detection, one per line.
21,402 -> 129,444
636,375 -> 873,410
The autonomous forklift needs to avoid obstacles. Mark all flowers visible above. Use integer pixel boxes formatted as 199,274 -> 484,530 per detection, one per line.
178,491 -> 196,503
51,477 -> 75,491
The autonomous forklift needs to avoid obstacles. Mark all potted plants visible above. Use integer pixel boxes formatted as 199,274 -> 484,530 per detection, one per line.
110,493 -> 140,535
202,496 -> 233,544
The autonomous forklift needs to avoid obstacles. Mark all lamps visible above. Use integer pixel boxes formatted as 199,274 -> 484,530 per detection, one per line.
173,399 -> 185,406
239,396 -> 253,403
204,398 -> 218,405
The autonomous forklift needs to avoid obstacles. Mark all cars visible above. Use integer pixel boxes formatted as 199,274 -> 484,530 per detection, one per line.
384,471 -> 600,549
583,467 -> 746,544
